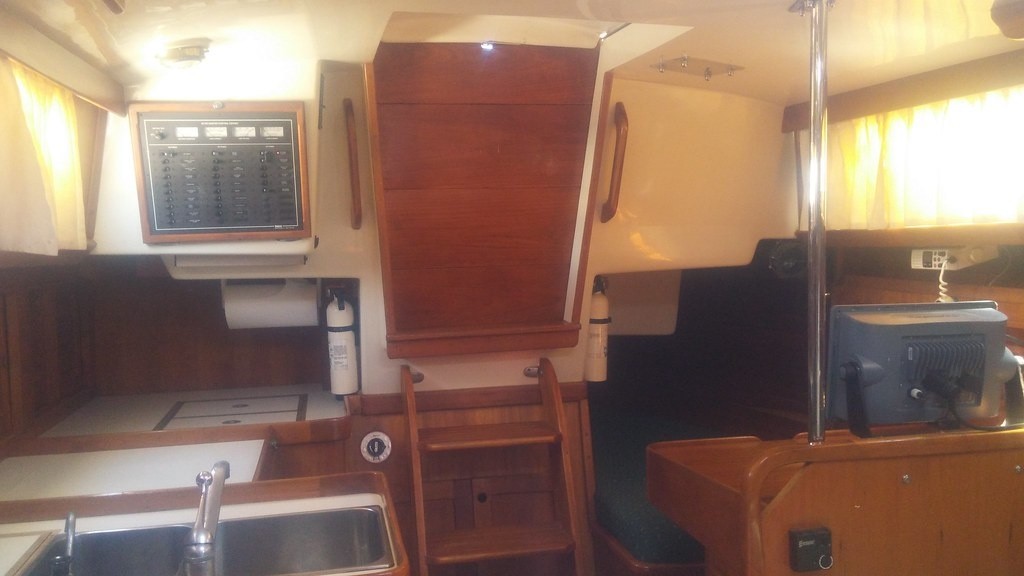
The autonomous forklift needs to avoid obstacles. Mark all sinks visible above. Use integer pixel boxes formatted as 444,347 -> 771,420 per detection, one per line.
23,504 -> 203,576
204,504 -> 395,576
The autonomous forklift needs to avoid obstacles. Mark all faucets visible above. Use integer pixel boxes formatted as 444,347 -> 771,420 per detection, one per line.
183,460 -> 231,576
64,511 -> 77,576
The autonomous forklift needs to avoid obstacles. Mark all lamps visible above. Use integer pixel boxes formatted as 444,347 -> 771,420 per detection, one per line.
826,300 -> 1007,438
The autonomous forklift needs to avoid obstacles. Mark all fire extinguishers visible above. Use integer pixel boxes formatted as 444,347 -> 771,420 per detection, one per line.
584,276 -> 610,382
323,280 -> 359,396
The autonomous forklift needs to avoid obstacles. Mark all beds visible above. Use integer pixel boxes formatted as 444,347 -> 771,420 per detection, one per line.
590,415 -> 735,576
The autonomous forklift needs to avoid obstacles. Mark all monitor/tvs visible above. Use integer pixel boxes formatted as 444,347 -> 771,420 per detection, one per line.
827,299 -> 1016,430
